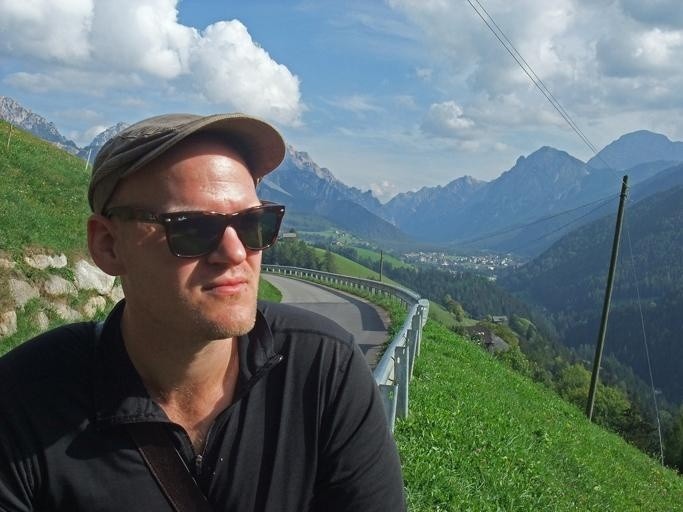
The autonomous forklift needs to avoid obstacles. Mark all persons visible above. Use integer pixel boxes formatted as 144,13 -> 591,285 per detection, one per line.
2,111 -> 407,511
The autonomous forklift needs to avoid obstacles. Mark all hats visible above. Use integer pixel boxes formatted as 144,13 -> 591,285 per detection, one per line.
87,112 -> 285,212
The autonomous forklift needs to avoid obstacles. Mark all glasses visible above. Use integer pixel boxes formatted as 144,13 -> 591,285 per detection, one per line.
102,202 -> 287,261
